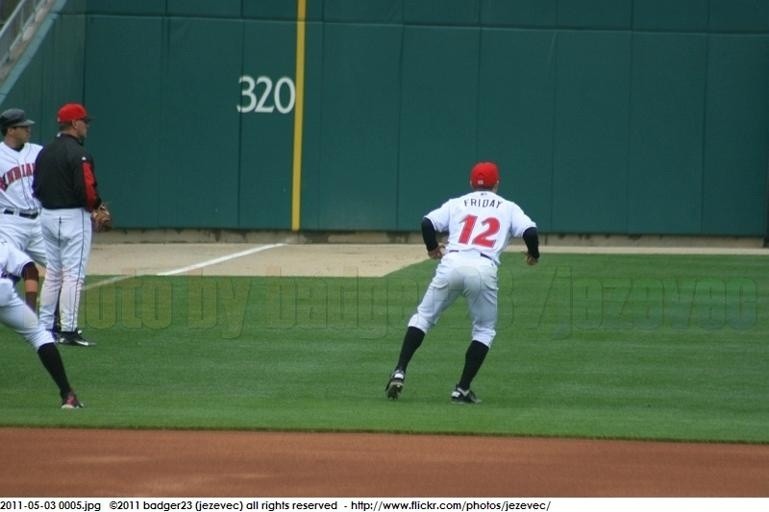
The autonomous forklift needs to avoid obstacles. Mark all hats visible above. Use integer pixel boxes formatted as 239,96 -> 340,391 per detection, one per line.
1,108 -> 35,130
470,161 -> 498,188
56,103 -> 96,123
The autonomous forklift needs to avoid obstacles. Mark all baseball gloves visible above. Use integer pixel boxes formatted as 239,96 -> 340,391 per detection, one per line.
91,203 -> 111,232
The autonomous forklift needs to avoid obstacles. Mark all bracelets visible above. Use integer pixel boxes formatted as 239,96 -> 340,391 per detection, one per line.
24,291 -> 38,312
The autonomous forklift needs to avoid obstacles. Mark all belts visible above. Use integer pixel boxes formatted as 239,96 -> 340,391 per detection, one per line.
4,209 -> 38,219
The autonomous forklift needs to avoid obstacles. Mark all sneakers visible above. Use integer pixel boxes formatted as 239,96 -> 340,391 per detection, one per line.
60,395 -> 84,409
450,387 -> 482,405
46,329 -> 90,346
385,369 -> 406,398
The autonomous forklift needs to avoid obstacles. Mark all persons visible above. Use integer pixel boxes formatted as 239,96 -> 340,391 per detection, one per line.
1,109 -> 83,335
0,233 -> 85,410
385,160 -> 541,404
31,103 -> 111,346
